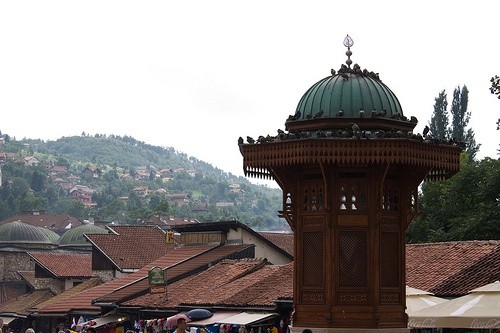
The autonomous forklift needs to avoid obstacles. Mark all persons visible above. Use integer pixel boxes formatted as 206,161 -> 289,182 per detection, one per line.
303,328 -> 312,333
1,324 -> 170,333
171,318 -> 190,333
